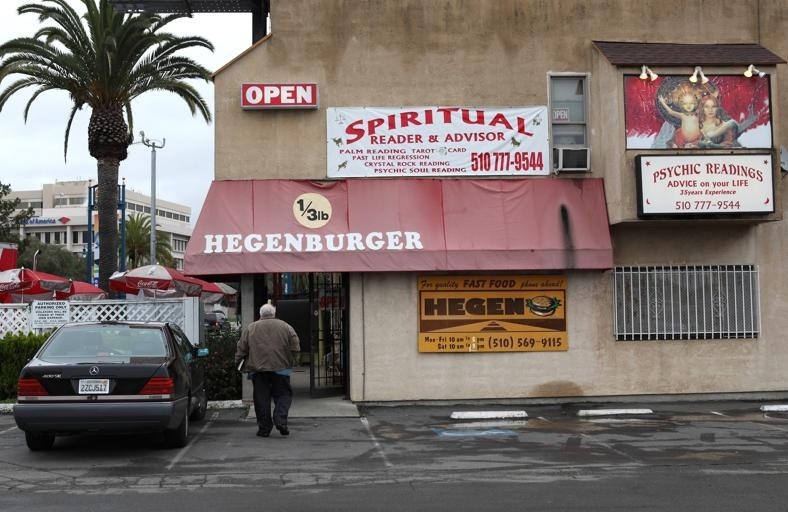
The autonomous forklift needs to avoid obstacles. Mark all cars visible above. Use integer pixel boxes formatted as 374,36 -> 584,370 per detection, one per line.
11,319 -> 211,451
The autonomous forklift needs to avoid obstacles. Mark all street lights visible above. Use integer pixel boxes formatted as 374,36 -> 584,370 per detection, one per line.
140,129 -> 167,265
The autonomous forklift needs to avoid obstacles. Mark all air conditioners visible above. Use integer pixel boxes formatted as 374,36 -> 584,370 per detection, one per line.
556,148 -> 590,172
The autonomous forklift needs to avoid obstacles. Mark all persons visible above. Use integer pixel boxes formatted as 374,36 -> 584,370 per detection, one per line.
656,90 -> 701,147
696,94 -> 737,149
233,302 -> 301,437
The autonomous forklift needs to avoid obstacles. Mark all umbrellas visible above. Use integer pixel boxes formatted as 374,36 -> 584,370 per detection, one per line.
0,266 -> 70,303
184,274 -> 236,303
12,279 -> 108,302
108,261 -> 201,299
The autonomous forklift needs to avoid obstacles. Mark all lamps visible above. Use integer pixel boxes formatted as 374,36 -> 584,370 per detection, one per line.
744,64 -> 765,79
689,66 -> 709,85
640,64 -> 659,81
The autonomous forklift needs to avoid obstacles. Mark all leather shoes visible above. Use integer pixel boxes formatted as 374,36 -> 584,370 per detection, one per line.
276,424 -> 290,436
256,431 -> 270,437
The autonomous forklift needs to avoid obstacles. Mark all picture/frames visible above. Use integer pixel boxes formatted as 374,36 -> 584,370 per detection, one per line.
623,73 -> 774,150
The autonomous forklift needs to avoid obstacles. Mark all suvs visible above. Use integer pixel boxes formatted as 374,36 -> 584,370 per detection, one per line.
204,310 -> 231,335
